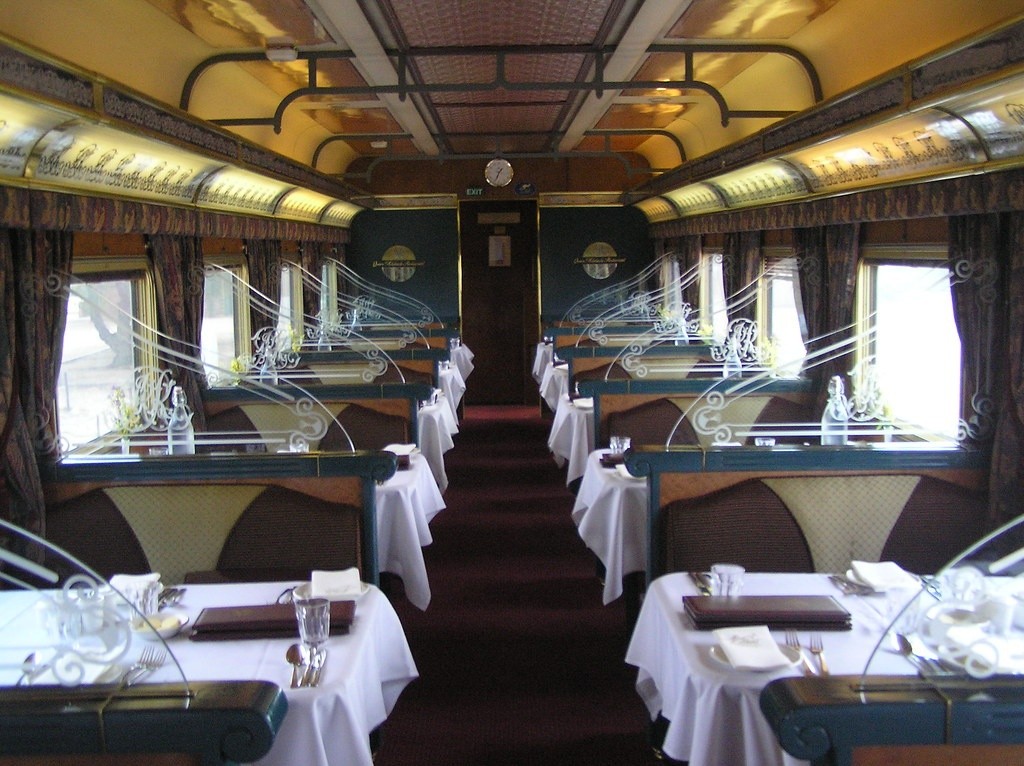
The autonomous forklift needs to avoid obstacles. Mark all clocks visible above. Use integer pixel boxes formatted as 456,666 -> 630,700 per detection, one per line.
484,157 -> 515,188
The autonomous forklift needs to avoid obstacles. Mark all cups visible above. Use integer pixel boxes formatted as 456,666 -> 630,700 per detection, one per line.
755,438 -> 775,446
290,444 -> 309,452
439,360 -> 449,371
611,436 -> 631,454
450,340 -> 459,348
554,352 -> 565,363
988,597 -> 1015,632
294,598 -> 330,649
97,586 -> 117,625
545,336 -> 553,341
423,388 -> 435,405
127,581 -> 158,616
149,447 -> 167,455
711,565 -> 745,595
75,596 -> 104,632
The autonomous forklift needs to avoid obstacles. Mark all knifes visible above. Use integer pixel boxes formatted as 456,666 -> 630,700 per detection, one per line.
309,649 -> 326,687
298,647 -> 316,687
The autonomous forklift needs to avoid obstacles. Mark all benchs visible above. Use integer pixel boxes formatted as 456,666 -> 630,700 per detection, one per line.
538,310 -> 988,587
41,314 -> 459,585
0,679 -> 287,766
759,675 -> 1024,766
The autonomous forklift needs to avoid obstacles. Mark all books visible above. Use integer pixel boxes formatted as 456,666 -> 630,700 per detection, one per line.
395,454 -> 411,472
682,593 -> 851,630
188,599 -> 357,641
599,454 -> 626,469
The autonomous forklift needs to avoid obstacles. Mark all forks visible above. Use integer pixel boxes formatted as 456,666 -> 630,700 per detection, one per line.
127,650 -> 167,687
810,633 -> 829,675
118,646 -> 156,687
786,628 -> 814,676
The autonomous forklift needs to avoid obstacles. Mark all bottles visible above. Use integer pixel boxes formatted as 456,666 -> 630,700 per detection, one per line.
168,386 -> 195,454
318,324 -> 331,352
260,346 -> 278,386
723,338 -> 742,378
821,377 -> 848,445
352,309 -> 362,331
675,318 -> 689,345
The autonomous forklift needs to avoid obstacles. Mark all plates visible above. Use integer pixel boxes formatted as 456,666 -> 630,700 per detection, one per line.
709,641 -> 802,670
293,583 -> 370,601
131,613 -> 189,640
926,601 -> 989,627
940,638 -> 1024,675
116,584 -> 163,605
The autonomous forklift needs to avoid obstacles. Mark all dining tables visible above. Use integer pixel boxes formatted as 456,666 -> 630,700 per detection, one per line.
625,565 -> 1024,766
533,341 -> 570,413
417,388 -> 459,496
550,391 -> 596,487
430,336 -> 474,455
373,440 -> 445,612
571,446 -> 647,604
0,577 -> 417,766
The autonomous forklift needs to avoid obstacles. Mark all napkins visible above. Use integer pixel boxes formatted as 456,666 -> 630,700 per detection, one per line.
615,464 -> 646,480
714,626 -> 790,671
307,568 -> 361,601
107,572 -> 160,610
851,560 -> 922,592
35,648 -> 116,683
381,443 -> 416,456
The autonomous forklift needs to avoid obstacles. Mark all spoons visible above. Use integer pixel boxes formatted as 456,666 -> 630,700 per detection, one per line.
891,634 -> 923,670
286,644 -> 303,688
157,588 -> 186,611
17,652 -> 40,685
